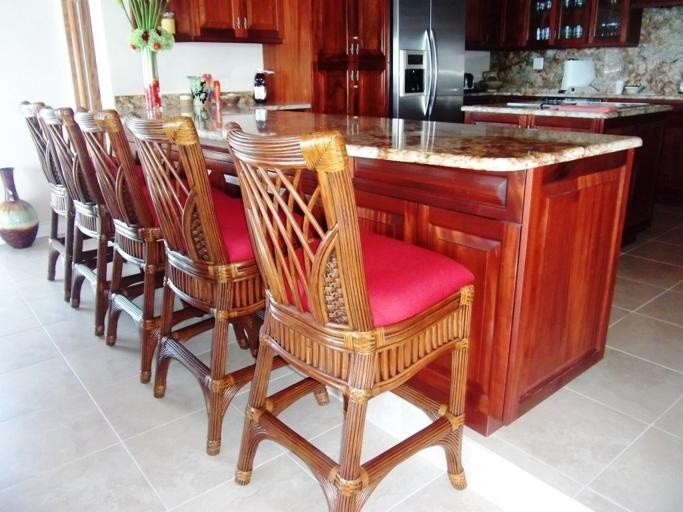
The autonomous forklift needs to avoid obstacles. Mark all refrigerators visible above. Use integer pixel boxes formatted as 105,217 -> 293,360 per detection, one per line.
391,0 -> 464,124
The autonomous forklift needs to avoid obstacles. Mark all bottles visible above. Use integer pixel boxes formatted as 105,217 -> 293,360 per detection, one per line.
536,0 -> 621,41
253,68 -> 267,105
216,109 -> 222,129
213,80 -> 221,109
254,109 -> 267,137
160,8 -> 176,36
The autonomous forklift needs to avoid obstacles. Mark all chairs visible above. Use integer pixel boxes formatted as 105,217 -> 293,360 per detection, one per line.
40,106 -> 164,338
221,120 -> 478,510
125,112 -> 335,459
19,99 -> 127,305
75,104 -> 250,385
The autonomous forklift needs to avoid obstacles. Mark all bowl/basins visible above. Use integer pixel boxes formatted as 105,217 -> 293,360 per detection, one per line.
623,87 -> 643,95
218,93 -> 241,108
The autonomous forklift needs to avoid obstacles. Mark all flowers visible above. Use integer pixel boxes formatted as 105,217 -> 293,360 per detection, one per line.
120,1 -> 177,51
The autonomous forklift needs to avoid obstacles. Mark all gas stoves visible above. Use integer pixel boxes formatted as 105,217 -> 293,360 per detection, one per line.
506,98 -> 651,111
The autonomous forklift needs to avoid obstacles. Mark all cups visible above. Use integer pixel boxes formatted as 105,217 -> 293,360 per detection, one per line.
192,108 -> 215,134
189,74 -> 212,107
181,108 -> 192,118
611,80 -> 624,96
179,95 -> 192,108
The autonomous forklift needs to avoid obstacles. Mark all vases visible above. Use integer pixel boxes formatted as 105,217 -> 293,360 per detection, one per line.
139,50 -> 165,120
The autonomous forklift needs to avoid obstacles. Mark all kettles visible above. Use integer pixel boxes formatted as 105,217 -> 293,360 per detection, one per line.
464,73 -> 475,92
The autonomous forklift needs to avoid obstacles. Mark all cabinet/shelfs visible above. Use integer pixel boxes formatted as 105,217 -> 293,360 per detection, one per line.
466,1 -> 641,53
460,93 -> 683,246
132,1 -> 392,117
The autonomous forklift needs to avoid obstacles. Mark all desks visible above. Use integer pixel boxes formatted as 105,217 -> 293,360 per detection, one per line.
97,109 -> 643,439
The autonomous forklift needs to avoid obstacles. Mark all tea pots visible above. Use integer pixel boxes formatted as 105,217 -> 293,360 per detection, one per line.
483,69 -> 500,81
